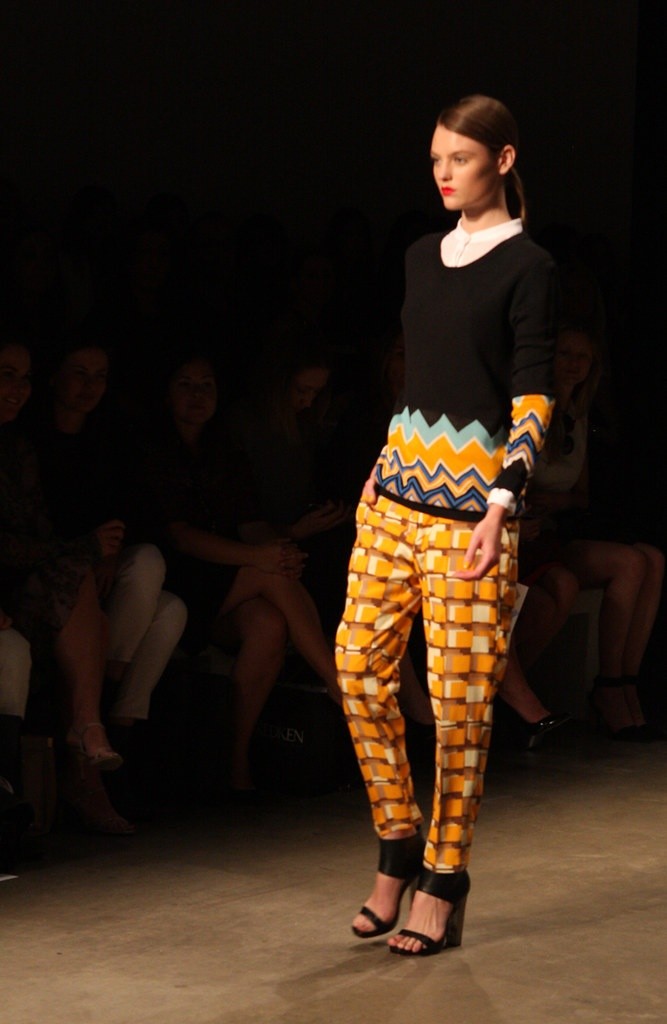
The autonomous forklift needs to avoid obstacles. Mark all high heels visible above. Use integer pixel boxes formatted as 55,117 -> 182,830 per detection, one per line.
351,824 -> 427,939
623,675 -> 657,742
389,868 -> 470,957
57,782 -> 136,834
588,675 -> 643,744
73,723 -> 124,781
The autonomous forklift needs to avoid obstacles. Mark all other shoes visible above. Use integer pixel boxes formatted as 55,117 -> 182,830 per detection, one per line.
0,799 -> 35,873
111,725 -> 132,776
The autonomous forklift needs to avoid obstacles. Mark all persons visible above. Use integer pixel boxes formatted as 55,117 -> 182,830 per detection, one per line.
335,93 -> 560,957
1,184 -> 665,850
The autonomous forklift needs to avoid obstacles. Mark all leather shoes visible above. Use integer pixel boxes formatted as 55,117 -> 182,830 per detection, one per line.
493,692 -> 574,736
404,713 -> 436,740
